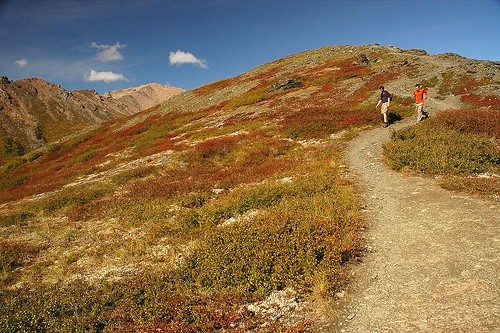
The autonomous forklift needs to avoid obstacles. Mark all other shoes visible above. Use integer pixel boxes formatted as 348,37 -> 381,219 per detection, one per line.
383,122 -> 389,127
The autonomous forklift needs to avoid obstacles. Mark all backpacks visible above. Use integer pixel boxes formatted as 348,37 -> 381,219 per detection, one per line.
384,90 -> 392,102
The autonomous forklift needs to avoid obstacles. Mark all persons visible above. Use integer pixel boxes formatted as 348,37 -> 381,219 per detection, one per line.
412,84 -> 429,122
375,86 -> 391,128
